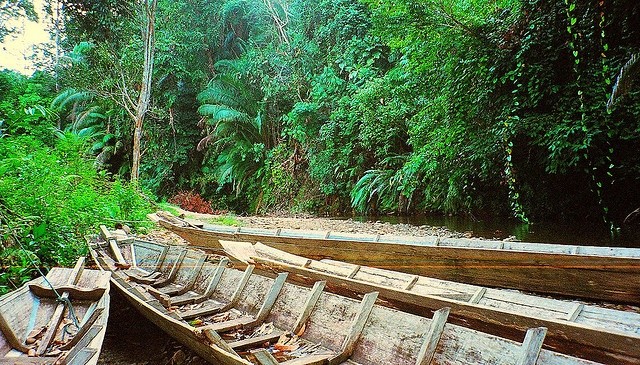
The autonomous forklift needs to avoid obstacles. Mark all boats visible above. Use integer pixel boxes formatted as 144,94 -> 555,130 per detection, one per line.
216,238 -> 640,365
83,228 -> 530,364
145,210 -> 640,306
0,255 -> 113,364
